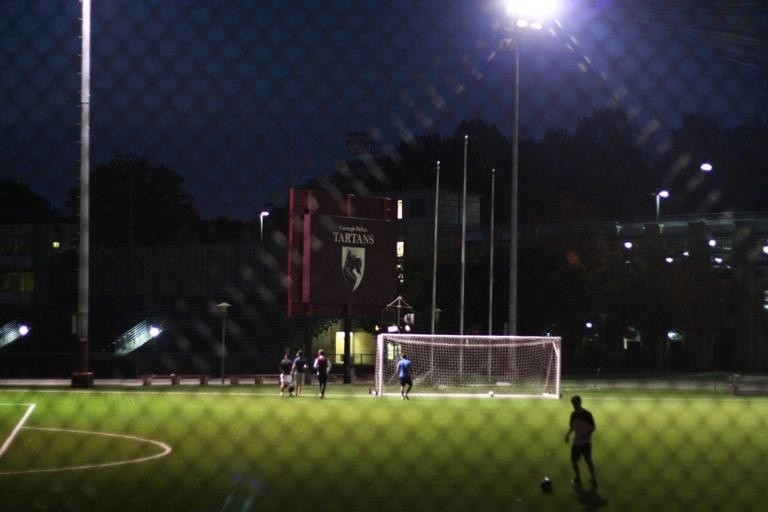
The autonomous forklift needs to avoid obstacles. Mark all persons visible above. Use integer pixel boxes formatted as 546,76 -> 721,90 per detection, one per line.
312,348 -> 332,398
289,350 -> 309,398
278,352 -> 295,398
395,353 -> 413,401
563,395 -> 597,485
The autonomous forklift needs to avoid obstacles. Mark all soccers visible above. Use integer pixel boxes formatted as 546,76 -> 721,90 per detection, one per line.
489,391 -> 495,397
540,476 -> 553,492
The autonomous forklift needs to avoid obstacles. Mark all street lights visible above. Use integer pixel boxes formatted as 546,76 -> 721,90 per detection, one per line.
260,210 -> 270,284
217,302 -> 232,385
506,0 -> 559,369
656,191 -> 669,222
700,162 -> 712,217
